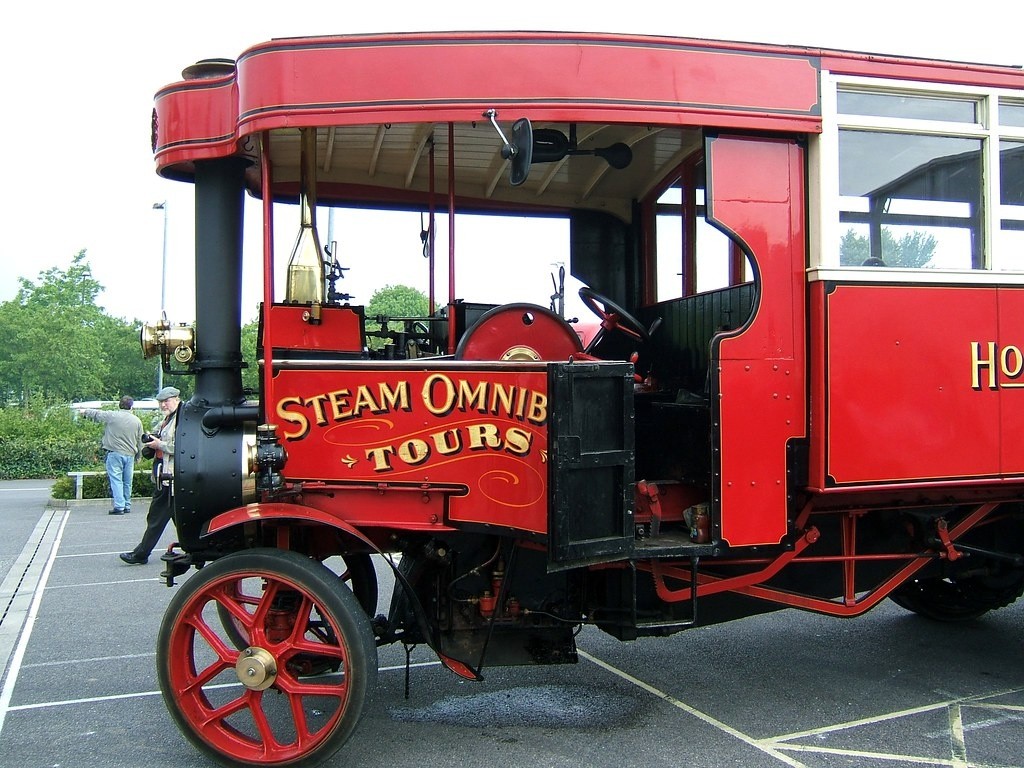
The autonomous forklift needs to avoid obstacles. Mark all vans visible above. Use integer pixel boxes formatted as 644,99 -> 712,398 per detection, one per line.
43,401 -> 159,422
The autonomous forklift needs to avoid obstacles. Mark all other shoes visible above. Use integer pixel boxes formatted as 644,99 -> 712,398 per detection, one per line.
125,509 -> 130,513
109,509 -> 124,514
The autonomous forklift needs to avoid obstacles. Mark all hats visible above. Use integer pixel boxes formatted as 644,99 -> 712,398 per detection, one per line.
156,387 -> 180,401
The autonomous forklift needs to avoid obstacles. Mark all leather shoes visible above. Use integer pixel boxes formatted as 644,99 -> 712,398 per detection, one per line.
120,552 -> 148,564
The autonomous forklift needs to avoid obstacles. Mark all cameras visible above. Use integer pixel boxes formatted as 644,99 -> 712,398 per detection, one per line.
141,433 -> 161,443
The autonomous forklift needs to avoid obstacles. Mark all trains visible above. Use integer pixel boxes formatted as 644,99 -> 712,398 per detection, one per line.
139,31 -> 1024,767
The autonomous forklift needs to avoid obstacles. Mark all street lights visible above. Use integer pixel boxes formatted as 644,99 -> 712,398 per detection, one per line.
151,201 -> 168,403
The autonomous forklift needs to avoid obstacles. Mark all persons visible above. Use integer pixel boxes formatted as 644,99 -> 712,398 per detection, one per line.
119,387 -> 180,565
77,395 -> 145,515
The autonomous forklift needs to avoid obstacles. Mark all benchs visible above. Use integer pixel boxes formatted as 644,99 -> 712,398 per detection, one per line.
68,470 -> 152,500
622,280 -> 755,538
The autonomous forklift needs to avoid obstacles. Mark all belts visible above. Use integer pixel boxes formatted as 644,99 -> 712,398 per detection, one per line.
108,450 -> 113,452
